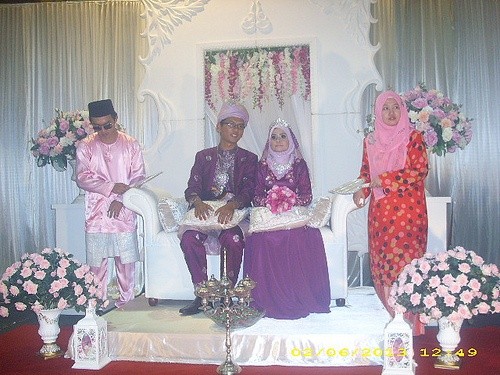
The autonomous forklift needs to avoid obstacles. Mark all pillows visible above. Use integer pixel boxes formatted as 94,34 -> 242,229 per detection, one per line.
248,206 -> 313,234
179,201 -> 249,230
306,196 -> 333,229
158,191 -> 187,233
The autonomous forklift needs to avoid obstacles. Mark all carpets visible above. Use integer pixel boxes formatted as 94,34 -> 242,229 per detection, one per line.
0,325 -> 500,375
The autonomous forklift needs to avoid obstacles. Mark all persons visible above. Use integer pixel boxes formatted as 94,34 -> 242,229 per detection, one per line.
353,91 -> 429,336
243,119 -> 331,320
75,99 -> 146,302
179,104 -> 259,314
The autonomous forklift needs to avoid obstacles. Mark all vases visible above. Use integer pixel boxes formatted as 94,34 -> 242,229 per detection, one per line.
433,315 -> 463,370
35,306 -> 66,360
68,159 -> 87,204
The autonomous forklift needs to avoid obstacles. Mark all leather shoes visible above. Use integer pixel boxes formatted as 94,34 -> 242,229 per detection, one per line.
179,297 -> 202,315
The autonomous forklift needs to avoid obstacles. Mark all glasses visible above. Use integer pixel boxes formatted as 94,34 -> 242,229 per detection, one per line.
222,121 -> 244,130
92,123 -> 114,130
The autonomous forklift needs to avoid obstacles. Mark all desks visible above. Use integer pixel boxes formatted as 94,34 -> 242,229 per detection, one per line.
52,204 -> 118,316
425,196 -> 452,254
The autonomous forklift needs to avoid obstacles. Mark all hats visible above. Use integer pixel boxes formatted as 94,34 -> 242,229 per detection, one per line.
88,99 -> 114,117
216,101 -> 249,127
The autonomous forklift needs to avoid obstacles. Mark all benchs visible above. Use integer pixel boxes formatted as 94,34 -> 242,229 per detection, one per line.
122,186 -> 370,308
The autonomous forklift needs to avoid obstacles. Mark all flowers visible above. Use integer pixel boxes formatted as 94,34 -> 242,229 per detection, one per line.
0,247 -> 110,319
28,108 -> 127,171
204,44 -> 311,113
355,80 -> 475,156
265,184 -> 299,216
388,244 -> 500,332
